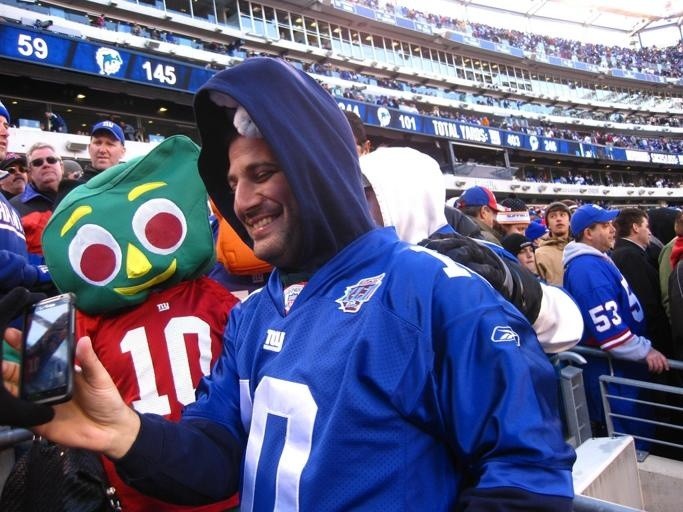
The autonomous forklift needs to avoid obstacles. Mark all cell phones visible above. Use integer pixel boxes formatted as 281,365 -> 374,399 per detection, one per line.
19,293 -> 77,405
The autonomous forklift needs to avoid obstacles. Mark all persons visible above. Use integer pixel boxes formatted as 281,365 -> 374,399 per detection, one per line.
533,201 -> 572,287
563,203 -> 669,463
77,119 -> 126,183
58,160 -> 84,182
358,146 -> 584,355
498,114 -> 554,140
244,48 -> 333,76
578,131 -> 683,157
447,111 -> 502,131
132,23 -> 228,55
658,212 -> 683,358
131,128 -> 168,144
413,91 -> 453,121
467,21 -> 610,68
668,211 -> 683,364
605,38 -> 683,79
341,110 -> 370,160
578,81 -> 683,128
454,186 -> 510,230
495,198 -> 531,239
314,80 -> 372,105
370,76 -> 418,114
344,0 -> 469,38
95,13 -> 105,26
502,233 -> 536,272
525,170 -> 596,185
1,58 -> 577,512
330,65 -> 373,86
9,143 -> 85,256
552,123 -> 580,143
43,111 -> 67,133
117,120 -> 136,141
525,209 -> 545,243
605,171 -> 683,190
610,209 -> 673,359
1,152 -> 28,200
0,103 -> 52,336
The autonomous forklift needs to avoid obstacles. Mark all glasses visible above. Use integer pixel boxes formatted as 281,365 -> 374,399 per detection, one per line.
31,156 -> 60,164
74,170 -> 85,180
4,168 -> 25,175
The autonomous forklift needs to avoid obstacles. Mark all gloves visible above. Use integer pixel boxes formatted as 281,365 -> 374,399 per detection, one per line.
0,286 -> 55,426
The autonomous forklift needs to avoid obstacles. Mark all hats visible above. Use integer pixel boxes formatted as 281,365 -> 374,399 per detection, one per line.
569,205 -> 619,233
2,151 -> 23,166
457,186 -> 507,211
495,198 -> 531,226
524,222 -> 547,239
543,202 -> 568,222
88,120 -> 124,145
501,234 -> 530,250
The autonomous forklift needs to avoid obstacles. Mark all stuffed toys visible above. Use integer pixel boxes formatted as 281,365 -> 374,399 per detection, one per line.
42,135 -> 243,511
